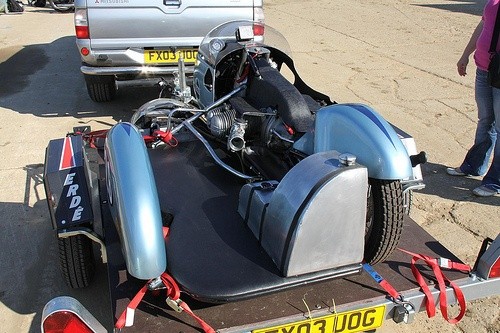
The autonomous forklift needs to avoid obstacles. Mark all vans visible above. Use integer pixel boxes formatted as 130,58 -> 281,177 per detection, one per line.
72,0 -> 265,104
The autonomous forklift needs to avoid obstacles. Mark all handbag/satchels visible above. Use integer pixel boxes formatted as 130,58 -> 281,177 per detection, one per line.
487,53 -> 500,89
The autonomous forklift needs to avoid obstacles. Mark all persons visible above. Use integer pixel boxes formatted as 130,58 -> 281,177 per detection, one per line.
446,0 -> 500,197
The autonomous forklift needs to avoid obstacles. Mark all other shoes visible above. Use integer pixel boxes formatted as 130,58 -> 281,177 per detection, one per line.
473,186 -> 497,197
445,167 -> 472,177
